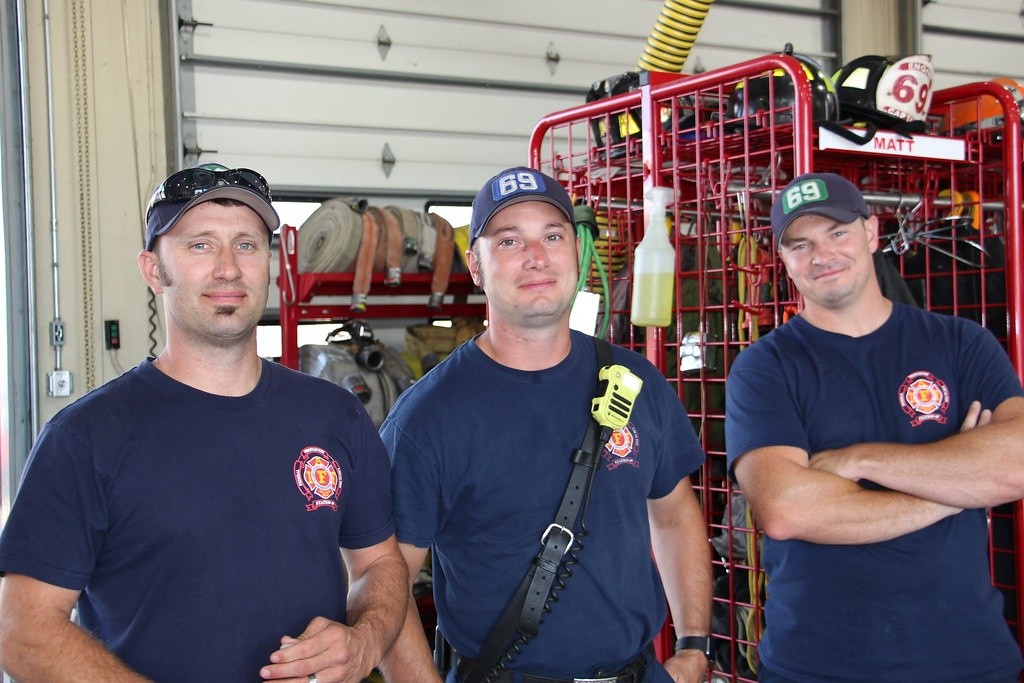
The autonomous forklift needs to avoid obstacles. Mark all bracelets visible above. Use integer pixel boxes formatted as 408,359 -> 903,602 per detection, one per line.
672,635 -> 714,662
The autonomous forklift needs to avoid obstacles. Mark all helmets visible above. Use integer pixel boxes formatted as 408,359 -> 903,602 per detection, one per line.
826,54 -> 934,132
727,41 -> 840,132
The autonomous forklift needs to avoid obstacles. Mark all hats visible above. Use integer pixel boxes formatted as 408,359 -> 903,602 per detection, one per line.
469,167 -> 578,249
145,163 -> 280,252
770,173 -> 869,253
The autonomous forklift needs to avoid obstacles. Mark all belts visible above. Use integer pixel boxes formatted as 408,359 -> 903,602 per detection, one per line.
450,641 -> 656,683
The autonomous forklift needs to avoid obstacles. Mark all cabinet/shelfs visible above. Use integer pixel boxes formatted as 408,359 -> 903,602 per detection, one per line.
526,53 -> 1024,683
275,225 -> 486,380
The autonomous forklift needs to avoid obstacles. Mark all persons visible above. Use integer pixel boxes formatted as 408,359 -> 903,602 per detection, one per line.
367,167 -> 714,683
725,172 -> 1024,682
2,161 -> 411,683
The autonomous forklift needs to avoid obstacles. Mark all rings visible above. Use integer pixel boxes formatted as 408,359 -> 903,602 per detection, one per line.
307,674 -> 318,683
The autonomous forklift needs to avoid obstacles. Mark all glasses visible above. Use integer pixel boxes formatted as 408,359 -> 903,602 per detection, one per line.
144,166 -> 272,222
